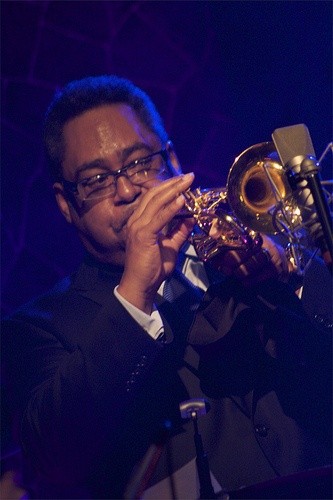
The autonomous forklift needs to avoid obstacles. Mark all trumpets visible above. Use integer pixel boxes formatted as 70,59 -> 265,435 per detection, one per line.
171,141 -> 311,262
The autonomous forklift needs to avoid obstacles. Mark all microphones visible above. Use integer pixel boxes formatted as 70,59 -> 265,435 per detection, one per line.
271,123 -> 332,272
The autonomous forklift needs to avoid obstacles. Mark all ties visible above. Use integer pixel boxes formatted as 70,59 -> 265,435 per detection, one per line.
155,254 -> 205,333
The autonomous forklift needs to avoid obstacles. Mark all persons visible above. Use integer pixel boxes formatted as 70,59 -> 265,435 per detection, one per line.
15,72 -> 333,499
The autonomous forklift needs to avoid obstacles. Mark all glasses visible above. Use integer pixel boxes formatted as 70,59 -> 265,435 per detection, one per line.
56,140 -> 169,202
